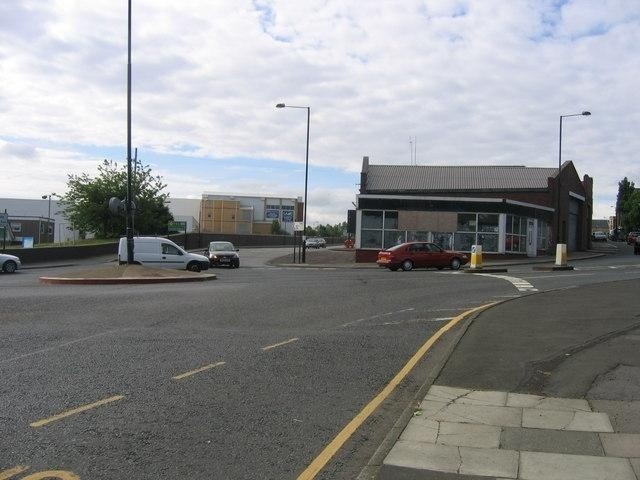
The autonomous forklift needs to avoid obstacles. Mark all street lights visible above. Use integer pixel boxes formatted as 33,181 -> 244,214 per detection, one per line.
42,195 -> 51,243
276,103 -> 310,264
555,111 -> 591,257
611,206 -> 617,239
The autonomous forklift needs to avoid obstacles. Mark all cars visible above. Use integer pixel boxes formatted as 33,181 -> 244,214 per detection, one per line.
607,227 -> 626,241
506,236 -> 525,251
345,236 -> 355,246
203,241 -> 240,268
625,231 -> 640,245
305,238 -> 320,249
376,241 -> 469,271
315,237 -> 327,249
0,253 -> 22,273
633,235 -> 640,255
591,231 -> 607,242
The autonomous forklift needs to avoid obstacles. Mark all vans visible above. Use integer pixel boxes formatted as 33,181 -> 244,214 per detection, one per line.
118,236 -> 210,273
